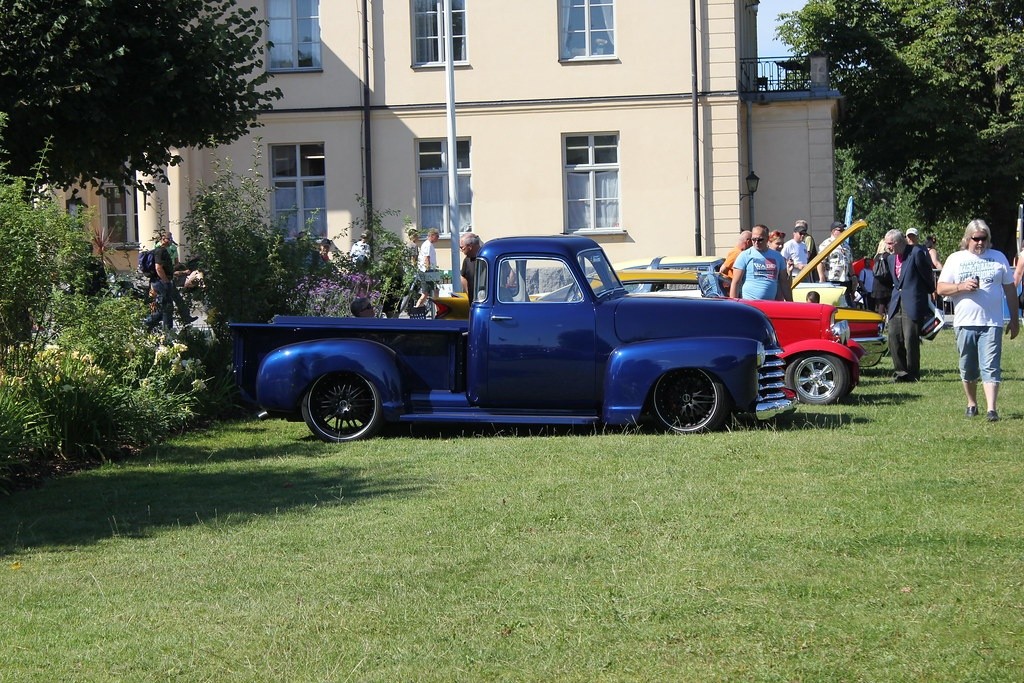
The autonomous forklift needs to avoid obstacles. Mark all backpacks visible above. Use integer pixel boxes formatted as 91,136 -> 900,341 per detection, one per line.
698,260 -> 731,298
138,248 -> 158,282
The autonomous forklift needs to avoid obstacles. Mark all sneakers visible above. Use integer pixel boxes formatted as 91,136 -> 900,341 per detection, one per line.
183,316 -> 199,327
987,410 -> 998,421
966,406 -> 978,417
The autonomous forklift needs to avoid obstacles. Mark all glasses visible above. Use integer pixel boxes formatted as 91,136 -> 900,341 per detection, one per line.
751,237 -> 764,242
971,234 -> 988,242
165,237 -> 173,241
838,229 -> 845,233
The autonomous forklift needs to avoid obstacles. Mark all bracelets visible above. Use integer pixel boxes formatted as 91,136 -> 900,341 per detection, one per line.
957,283 -> 961,294
851,274 -> 856,277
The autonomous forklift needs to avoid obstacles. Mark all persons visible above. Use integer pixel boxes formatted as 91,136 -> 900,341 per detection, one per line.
403,228 -> 440,306
459,233 -> 516,309
296,232 -> 338,275
720,220 -> 857,303
852,228 -> 943,383
144,232 -> 198,335
350,297 -> 375,317
83,241 -> 109,303
937,219 -> 1019,422
1013,246 -> 1024,317
350,230 -> 373,270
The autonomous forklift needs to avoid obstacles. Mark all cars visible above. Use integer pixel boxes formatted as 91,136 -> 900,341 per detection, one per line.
427,269 -> 891,406
530,253 -> 865,315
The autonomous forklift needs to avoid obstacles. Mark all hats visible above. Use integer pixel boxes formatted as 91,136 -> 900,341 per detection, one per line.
360,230 -> 373,240
794,226 -> 807,235
906,228 -> 919,236
832,220 -> 847,228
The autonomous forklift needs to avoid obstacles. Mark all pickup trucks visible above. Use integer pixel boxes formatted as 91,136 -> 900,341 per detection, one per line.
230,235 -> 800,444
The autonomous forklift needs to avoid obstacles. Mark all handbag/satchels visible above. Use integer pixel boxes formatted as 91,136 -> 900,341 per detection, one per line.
873,252 -> 893,289
